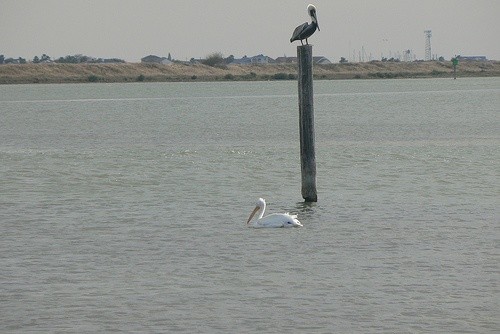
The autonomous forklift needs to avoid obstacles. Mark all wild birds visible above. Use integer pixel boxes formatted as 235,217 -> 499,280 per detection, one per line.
246,197 -> 303,228
290,3 -> 321,47
